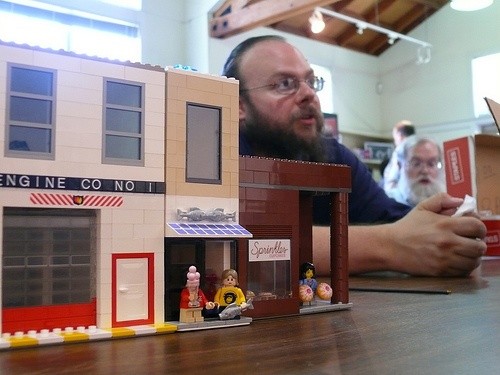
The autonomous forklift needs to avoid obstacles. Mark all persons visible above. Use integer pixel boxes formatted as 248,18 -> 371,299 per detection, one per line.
214,269 -> 247,320
222,36 -> 487,277
299,262 -> 318,305
179,266 -> 214,323
375,119 -> 446,208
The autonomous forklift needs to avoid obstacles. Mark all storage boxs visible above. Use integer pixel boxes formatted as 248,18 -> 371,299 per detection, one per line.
443,96 -> 500,260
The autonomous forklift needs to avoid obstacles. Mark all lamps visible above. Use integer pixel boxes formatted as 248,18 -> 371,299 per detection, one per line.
449,0 -> 495,13
309,8 -> 327,35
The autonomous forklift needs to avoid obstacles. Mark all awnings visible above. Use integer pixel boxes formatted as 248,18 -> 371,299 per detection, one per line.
167,223 -> 252,237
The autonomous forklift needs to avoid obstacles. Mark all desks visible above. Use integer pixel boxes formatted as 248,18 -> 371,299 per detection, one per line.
0,260 -> 500,375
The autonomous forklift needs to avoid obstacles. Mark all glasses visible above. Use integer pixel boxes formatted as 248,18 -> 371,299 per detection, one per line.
239,76 -> 325,95
